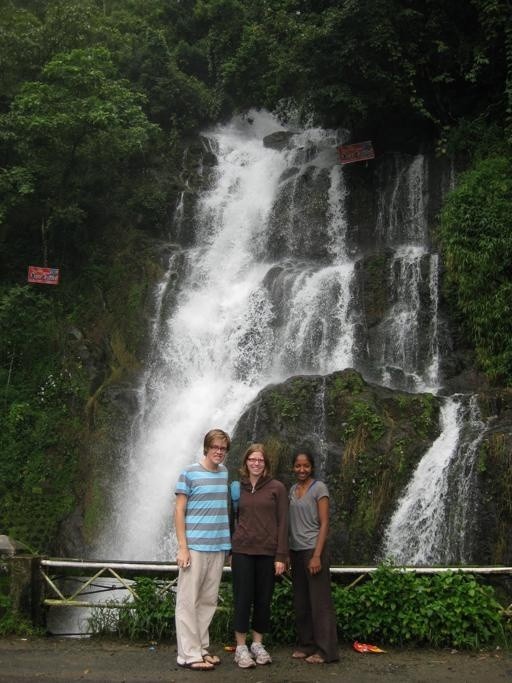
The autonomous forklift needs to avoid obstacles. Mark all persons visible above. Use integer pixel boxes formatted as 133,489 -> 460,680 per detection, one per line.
228,443 -> 288,669
288,447 -> 339,664
174,428 -> 231,670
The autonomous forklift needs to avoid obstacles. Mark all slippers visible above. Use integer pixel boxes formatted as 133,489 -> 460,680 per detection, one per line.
291,650 -> 327,664
177,654 -> 220,671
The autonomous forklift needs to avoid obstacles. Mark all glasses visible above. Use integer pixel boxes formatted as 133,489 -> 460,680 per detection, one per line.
247,459 -> 265,462
208,446 -> 227,451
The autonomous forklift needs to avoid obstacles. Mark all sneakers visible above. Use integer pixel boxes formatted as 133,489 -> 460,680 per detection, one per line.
234,643 -> 272,668
